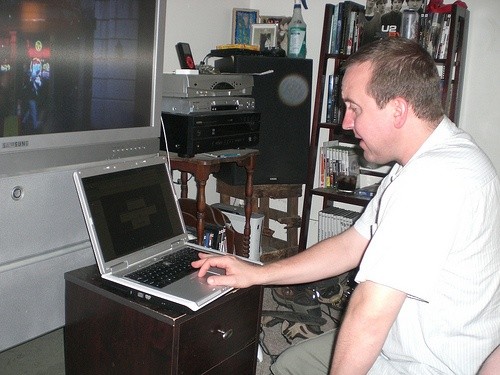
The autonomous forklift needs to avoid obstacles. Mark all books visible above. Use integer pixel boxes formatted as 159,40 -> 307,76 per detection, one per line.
186,224 -> 228,254
327,1 -> 366,57
318,146 -> 382,197
319,56 -> 345,126
417,12 -> 462,81
318,206 -> 362,245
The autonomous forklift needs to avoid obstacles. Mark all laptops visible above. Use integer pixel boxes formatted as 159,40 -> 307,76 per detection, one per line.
72,156 -> 234,312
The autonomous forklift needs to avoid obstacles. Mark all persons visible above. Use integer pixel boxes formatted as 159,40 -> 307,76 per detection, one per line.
16,87 -> 47,135
191,36 -> 500,375
361,0 -> 424,50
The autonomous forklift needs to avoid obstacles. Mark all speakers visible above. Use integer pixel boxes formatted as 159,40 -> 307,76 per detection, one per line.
214,54 -> 313,187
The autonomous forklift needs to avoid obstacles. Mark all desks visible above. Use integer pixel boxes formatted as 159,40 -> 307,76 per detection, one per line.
166,147 -> 261,259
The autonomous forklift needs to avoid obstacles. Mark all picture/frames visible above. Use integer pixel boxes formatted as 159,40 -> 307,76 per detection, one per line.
231,8 -> 259,46
249,22 -> 276,50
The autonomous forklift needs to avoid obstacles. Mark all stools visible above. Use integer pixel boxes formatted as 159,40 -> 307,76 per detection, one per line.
216,178 -> 303,264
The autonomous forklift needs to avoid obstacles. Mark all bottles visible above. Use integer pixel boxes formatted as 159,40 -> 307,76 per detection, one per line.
288,0 -> 308,58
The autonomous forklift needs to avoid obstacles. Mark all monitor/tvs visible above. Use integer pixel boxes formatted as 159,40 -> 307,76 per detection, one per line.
0,0 -> 167,177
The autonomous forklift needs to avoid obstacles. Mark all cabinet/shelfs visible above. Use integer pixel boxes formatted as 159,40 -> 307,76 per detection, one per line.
293,0 -> 470,254
63,263 -> 264,375
